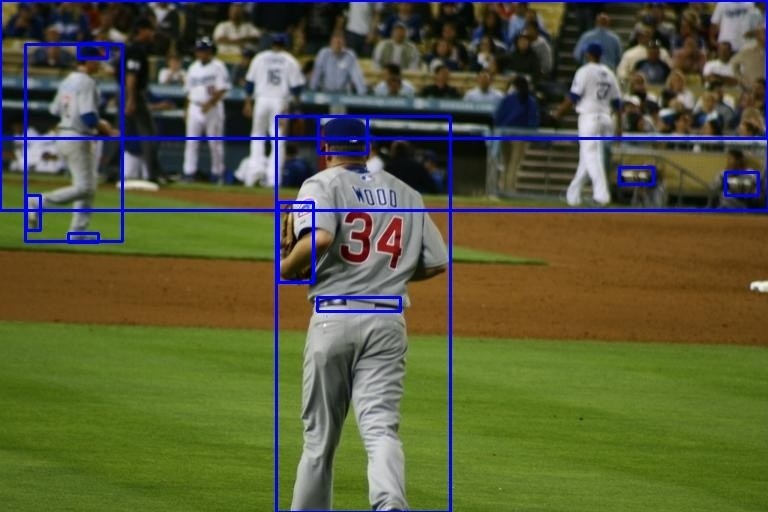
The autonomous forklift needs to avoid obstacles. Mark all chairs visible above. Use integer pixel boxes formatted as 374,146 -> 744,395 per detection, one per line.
69,233 -> 99,243
0,0 -> 768,138
26,195 -> 41,231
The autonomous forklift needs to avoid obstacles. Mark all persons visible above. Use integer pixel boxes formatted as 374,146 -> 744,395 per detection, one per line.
276,116 -> 450,512
26,45 -> 122,242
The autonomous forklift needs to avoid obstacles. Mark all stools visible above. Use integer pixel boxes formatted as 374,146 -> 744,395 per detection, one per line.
317,298 -> 401,311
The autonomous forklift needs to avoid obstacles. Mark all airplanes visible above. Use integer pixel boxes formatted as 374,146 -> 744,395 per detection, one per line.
278,203 -> 314,282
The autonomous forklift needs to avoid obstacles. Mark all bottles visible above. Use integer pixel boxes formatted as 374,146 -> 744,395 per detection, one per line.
79,44 -> 108,58
318,116 -> 367,154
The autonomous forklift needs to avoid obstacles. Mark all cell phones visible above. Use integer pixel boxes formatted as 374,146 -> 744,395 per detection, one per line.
619,168 -> 653,185
726,173 -> 758,195
